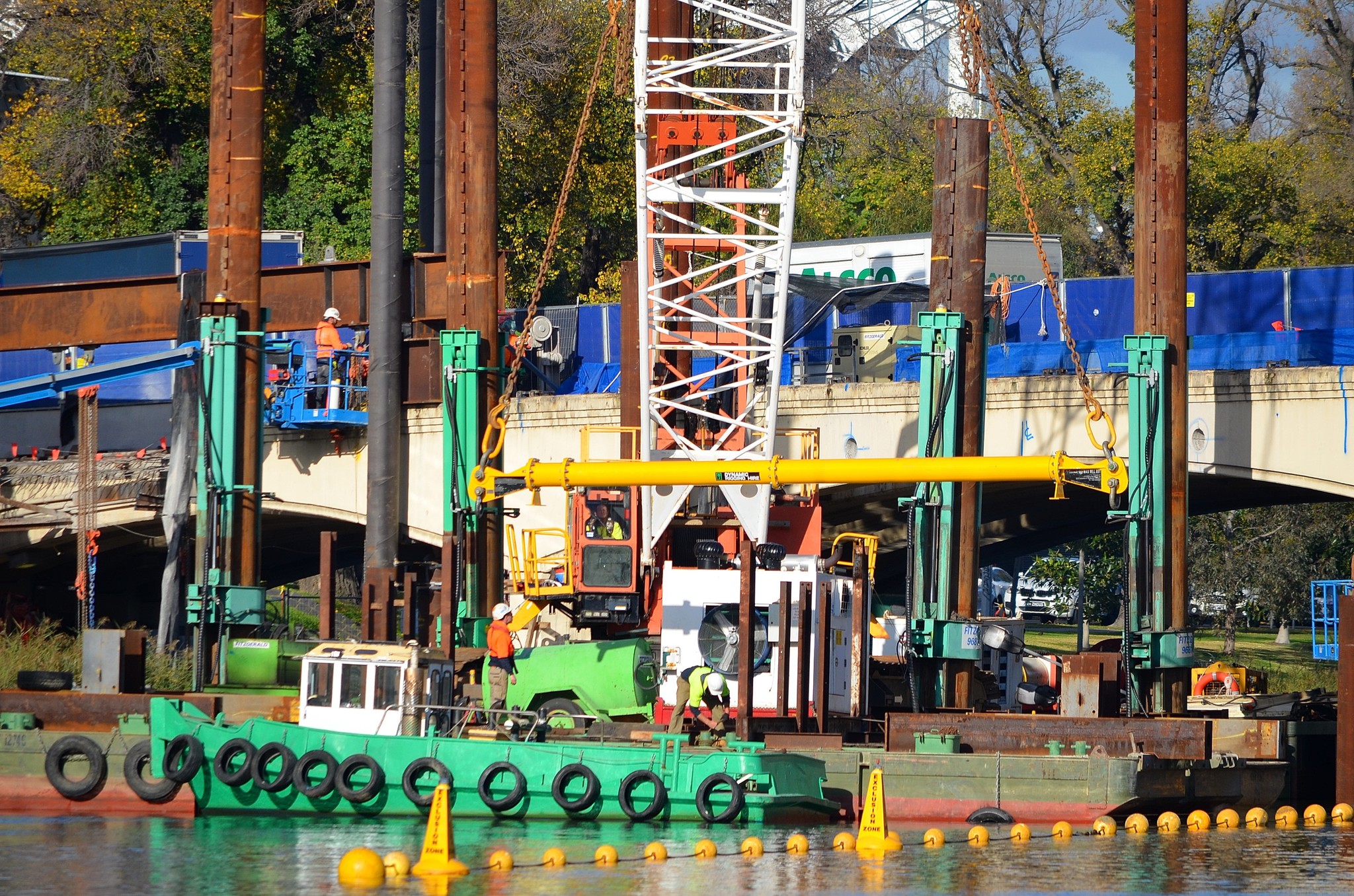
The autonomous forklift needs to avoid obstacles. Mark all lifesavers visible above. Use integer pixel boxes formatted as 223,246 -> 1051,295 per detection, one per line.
251,742 -> 298,794
695,772 -> 745,824
401,757 -> 454,808
163,734 -> 203,784
44,735 -> 107,798
618,770 -> 668,821
16,670 -> 74,692
214,738 -> 258,787
1192,671 -> 1240,696
123,739 -> 182,804
293,749 -> 340,798
335,754 -> 385,804
551,763 -> 601,813
477,762 -> 527,811
964,807 -> 1015,824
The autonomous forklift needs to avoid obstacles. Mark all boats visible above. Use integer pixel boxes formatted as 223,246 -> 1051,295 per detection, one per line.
0,538 -> 1290,824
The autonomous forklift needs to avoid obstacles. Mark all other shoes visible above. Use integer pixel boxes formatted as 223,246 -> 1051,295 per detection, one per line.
710,739 -> 727,747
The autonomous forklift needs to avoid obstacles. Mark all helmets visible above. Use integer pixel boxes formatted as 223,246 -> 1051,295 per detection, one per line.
491,603 -> 511,621
324,307 -> 343,322
707,672 -> 724,696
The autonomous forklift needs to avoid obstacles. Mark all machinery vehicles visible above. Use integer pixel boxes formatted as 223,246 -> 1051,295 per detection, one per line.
466,0 -> 1127,636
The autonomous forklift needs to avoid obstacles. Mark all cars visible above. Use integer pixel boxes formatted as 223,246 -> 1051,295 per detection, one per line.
975,557 -> 1345,629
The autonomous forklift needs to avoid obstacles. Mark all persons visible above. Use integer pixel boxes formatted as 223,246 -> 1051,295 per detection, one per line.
314,308 -> 352,410
498,320 -> 533,374
487,602 -> 518,713
669,665 -> 730,740
589,505 -> 623,542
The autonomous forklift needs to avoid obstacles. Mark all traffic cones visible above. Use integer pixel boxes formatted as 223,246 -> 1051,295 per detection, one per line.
853,768 -> 887,851
408,785 -> 468,874
